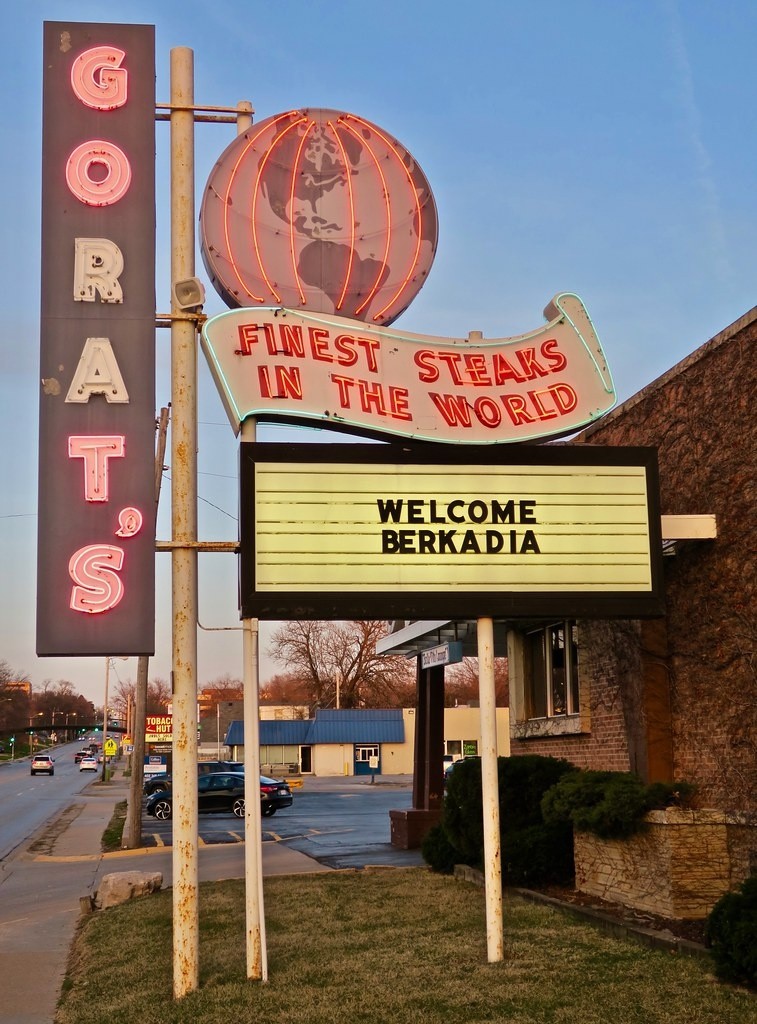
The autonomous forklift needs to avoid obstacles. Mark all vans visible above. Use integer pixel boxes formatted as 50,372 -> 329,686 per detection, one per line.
444,756 -> 483,793
89,745 -> 98,753
31,756 -> 55,776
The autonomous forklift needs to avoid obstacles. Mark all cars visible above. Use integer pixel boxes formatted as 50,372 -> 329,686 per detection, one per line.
80,758 -> 98,772
97,742 -> 103,749
145,762 -> 244,795
98,755 -> 110,764
146,772 -> 293,820
78,733 -> 119,741
74,747 -> 94,763
